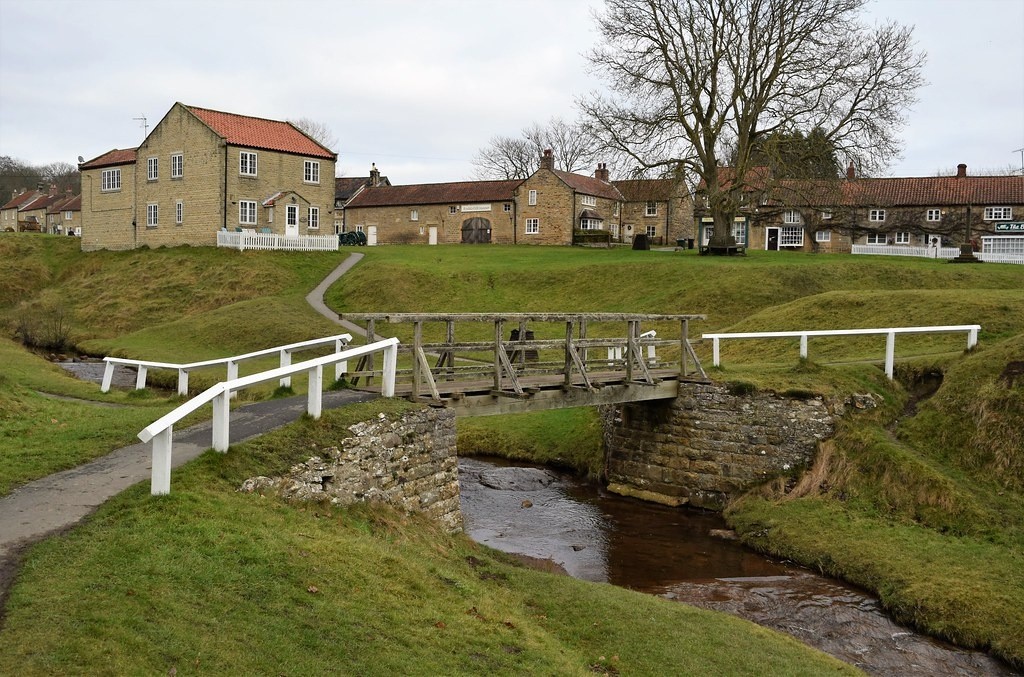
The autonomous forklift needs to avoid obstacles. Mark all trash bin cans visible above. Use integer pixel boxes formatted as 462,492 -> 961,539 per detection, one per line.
677,238 -> 687,250
688,239 -> 695,249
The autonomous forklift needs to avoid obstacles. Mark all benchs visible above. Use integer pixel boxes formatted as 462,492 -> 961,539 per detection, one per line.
699,236 -> 745,255
